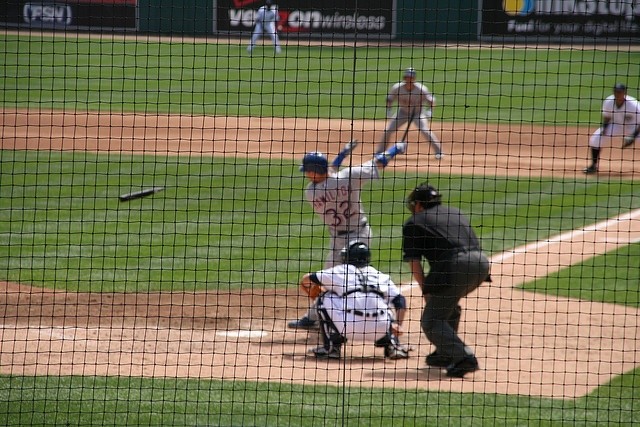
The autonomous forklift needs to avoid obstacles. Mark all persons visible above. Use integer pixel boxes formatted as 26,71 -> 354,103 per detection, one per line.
245,1 -> 282,54
400,182 -> 492,380
582,81 -> 640,174
287,138 -> 408,329
372,67 -> 443,159
300,239 -> 410,359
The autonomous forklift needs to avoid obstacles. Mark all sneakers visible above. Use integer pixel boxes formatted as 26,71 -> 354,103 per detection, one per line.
289,317 -> 316,328
426,350 -> 455,366
388,340 -> 409,359
312,346 -> 340,358
447,356 -> 478,378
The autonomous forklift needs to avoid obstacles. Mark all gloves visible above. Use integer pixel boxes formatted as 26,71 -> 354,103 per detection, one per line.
374,142 -> 408,166
334,139 -> 358,169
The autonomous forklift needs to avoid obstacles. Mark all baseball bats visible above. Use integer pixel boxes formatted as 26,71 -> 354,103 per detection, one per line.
119,184 -> 166,201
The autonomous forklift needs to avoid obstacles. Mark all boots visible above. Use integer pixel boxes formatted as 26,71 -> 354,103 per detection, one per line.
583,146 -> 600,174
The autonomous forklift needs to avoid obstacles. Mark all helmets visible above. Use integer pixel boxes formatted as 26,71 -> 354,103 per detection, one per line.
402,67 -> 416,79
405,184 -> 441,209
339,241 -> 370,267
301,152 -> 328,174
